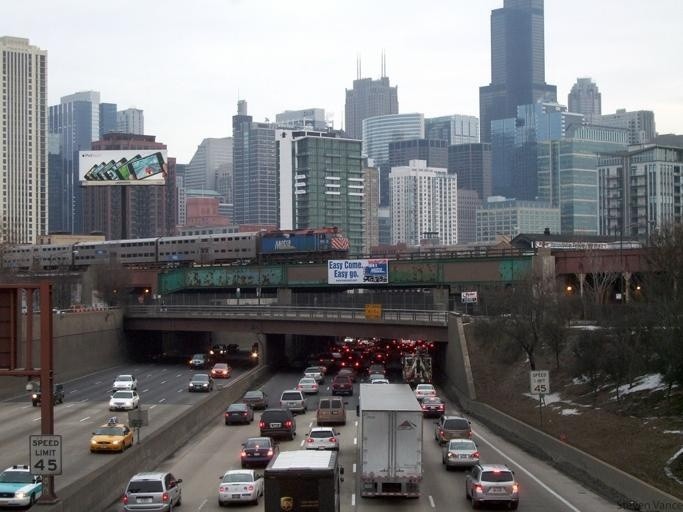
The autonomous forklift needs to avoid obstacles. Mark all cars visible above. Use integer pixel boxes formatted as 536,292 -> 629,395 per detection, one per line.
185,336 -> 522,511
1,461 -> 43,508
118,470 -> 184,512
111,374 -> 139,392
108,388 -> 141,414
19,301 -> 107,315
87,415 -> 134,454
29,383 -> 68,406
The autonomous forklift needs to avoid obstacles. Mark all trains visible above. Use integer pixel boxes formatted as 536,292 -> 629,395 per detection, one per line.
0,226 -> 352,273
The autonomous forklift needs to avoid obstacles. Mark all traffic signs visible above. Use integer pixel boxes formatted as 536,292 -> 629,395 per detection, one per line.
29,433 -> 63,475
530,370 -> 550,395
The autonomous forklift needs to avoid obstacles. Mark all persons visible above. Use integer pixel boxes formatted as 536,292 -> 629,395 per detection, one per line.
161,300 -> 167,312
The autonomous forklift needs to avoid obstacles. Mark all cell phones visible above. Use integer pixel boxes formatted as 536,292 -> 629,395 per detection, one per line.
84,152 -> 165,181
